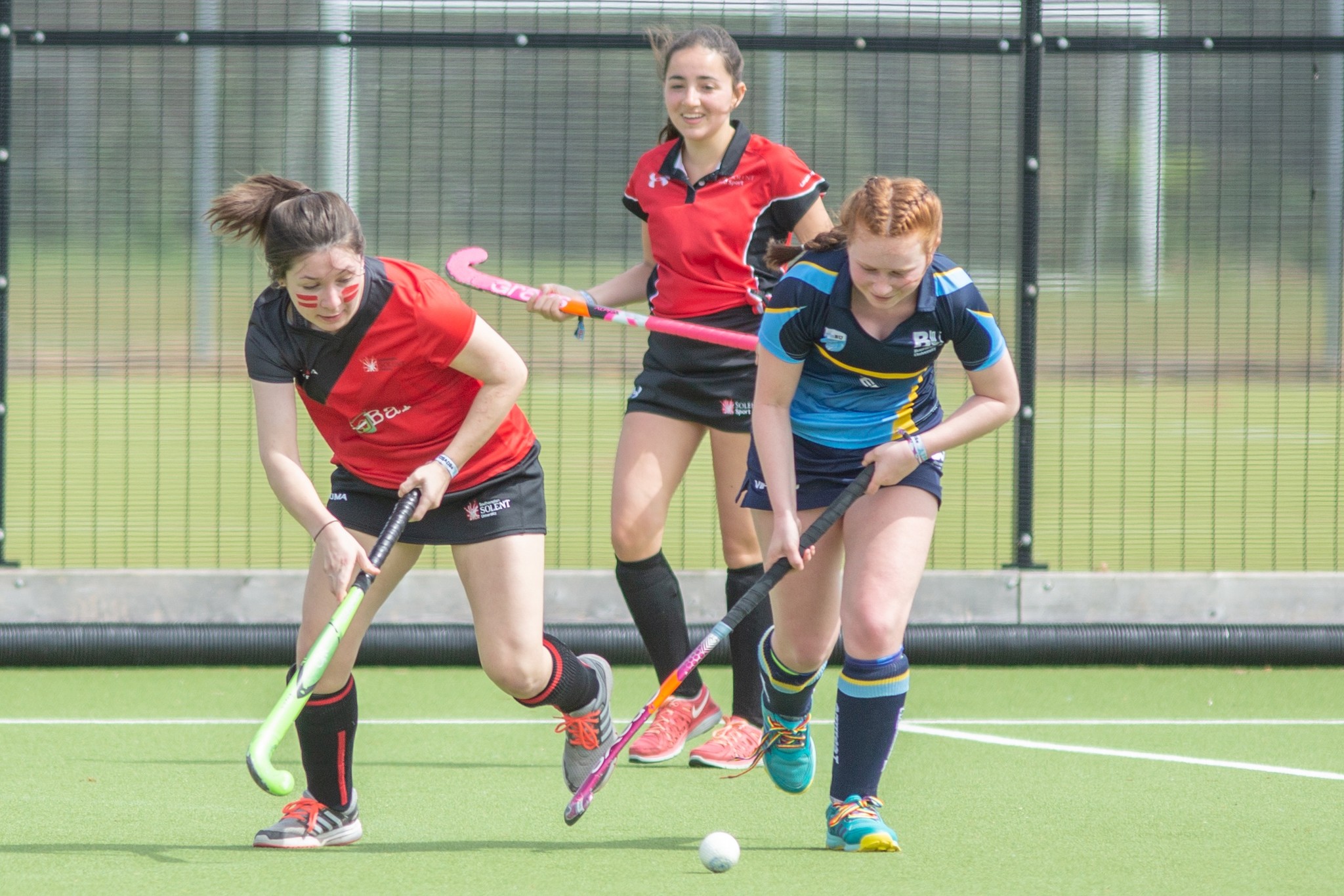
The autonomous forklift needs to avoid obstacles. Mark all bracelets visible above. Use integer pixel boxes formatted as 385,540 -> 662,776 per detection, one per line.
579,290 -> 597,305
314,520 -> 339,541
435,454 -> 459,478
898,428 -> 922,464
911,435 -> 927,461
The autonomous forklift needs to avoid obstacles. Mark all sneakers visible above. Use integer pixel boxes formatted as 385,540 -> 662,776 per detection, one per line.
825,793 -> 903,853
718,689 -> 816,794
552,654 -> 618,794
628,682 -> 722,764
253,787 -> 364,847
689,715 -> 765,770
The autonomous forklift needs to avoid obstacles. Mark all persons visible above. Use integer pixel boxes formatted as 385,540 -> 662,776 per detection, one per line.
522,16 -> 834,772
203,172 -> 617,847
733,175 -> 1020,852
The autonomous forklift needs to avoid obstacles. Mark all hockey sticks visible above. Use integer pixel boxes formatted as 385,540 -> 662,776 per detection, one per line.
562,466 -> 878,827
242,487 -> 417,799
446,243 -> 755,355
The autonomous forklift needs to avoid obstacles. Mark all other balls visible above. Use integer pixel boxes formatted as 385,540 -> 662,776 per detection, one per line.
698,829 -> 742,873
